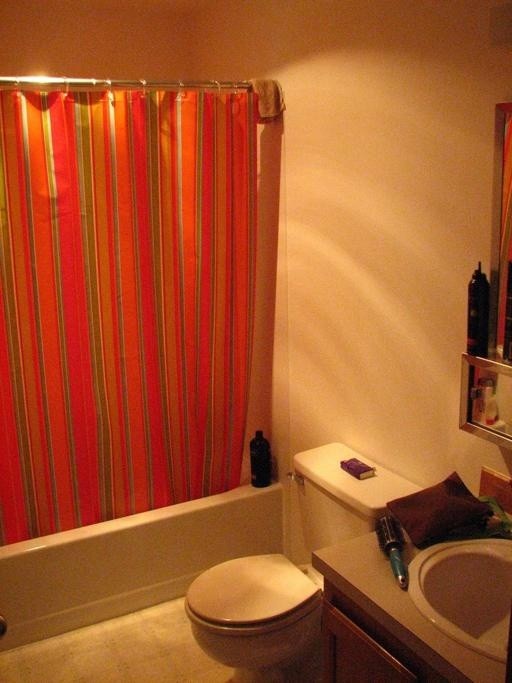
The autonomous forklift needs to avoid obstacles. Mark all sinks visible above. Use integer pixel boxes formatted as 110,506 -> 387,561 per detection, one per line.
407,538 -> 512,663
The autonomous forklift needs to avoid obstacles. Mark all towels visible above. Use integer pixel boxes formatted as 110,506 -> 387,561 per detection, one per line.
385,467 -> 495,546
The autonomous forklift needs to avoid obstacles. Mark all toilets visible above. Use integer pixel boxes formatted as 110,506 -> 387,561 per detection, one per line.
184,440 -> 429,670
